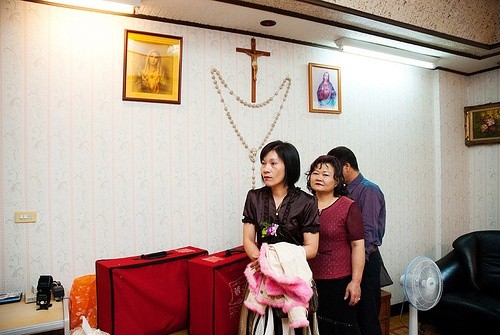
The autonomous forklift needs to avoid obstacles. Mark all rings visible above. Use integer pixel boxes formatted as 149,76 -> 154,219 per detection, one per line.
359,298 -> 360,300
257,268 -> 260,271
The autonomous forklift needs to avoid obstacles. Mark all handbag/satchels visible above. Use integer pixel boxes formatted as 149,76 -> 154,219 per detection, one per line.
187,242 -> 258,335
94,246 -> 209,335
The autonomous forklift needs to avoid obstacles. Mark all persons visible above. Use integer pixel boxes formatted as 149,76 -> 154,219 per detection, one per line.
305,155 -> 367,335
327,146 -> 386,335
240,140 -> 322,335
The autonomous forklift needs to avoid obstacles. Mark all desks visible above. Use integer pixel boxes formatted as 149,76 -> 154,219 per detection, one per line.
0,295 -> 71,335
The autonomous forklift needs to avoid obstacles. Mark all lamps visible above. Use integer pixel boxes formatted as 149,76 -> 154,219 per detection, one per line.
42,0 -> 142,15
334,37 -> 441,69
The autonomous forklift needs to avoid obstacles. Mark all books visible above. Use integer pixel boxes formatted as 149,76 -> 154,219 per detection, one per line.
0,293 -> 22,304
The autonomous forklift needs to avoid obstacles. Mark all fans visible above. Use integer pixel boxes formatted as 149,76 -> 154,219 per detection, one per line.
400,256 -> 443,335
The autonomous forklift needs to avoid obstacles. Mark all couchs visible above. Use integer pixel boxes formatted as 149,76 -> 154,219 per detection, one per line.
420,230 -> 500,335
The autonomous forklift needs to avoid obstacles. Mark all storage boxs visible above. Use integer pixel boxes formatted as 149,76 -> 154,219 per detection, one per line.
96,242 -> 258,335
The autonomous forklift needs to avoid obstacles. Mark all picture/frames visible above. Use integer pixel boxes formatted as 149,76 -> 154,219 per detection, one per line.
122,29 -> 183,105
464,102 -> 500,147
308,63 -> 342,114
